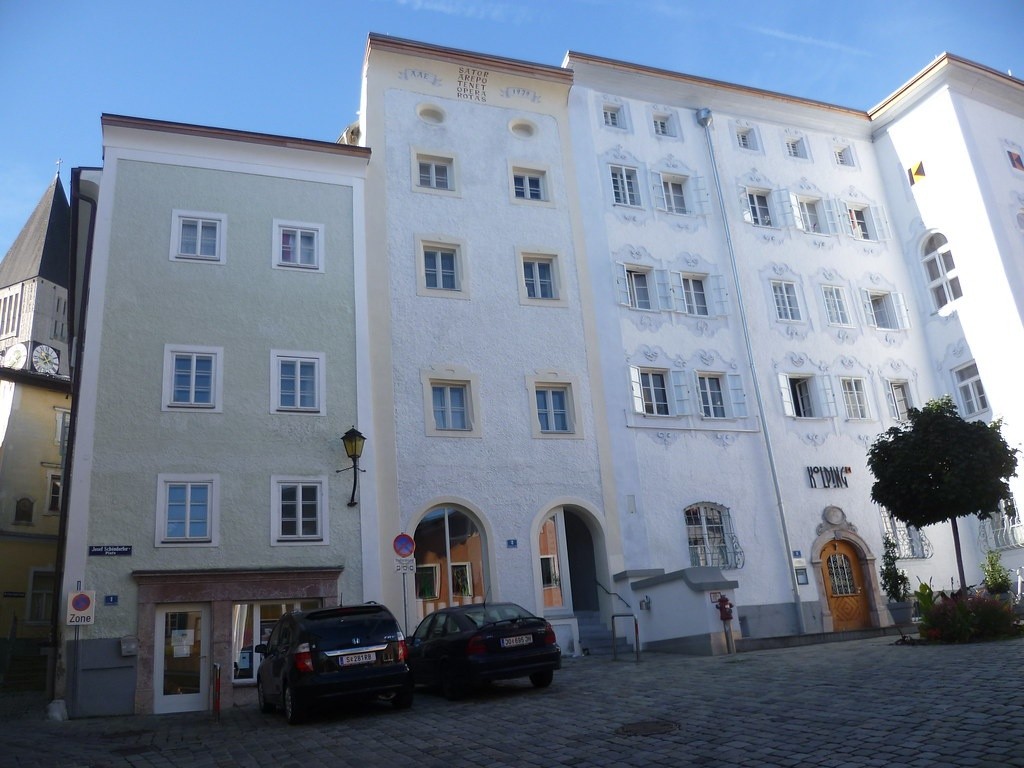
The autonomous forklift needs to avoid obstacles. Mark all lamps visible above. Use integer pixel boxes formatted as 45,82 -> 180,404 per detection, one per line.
336,425 -> 367,506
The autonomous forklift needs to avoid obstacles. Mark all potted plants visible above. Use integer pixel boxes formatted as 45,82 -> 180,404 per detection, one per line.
879,534 -> 912,624
980,548 -> 1015,606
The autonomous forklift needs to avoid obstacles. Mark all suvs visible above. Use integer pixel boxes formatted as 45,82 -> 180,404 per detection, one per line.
254,601 -> 415,725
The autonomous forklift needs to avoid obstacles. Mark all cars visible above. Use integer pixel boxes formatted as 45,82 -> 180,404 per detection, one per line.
403,601 -> 562,698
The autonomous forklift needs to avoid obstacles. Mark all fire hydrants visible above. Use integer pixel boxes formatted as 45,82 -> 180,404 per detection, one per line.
716,594 -> 735,621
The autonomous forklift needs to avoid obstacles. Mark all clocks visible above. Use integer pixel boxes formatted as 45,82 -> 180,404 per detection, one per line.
30,340 -> 61,374
3,340 -> 29,370
